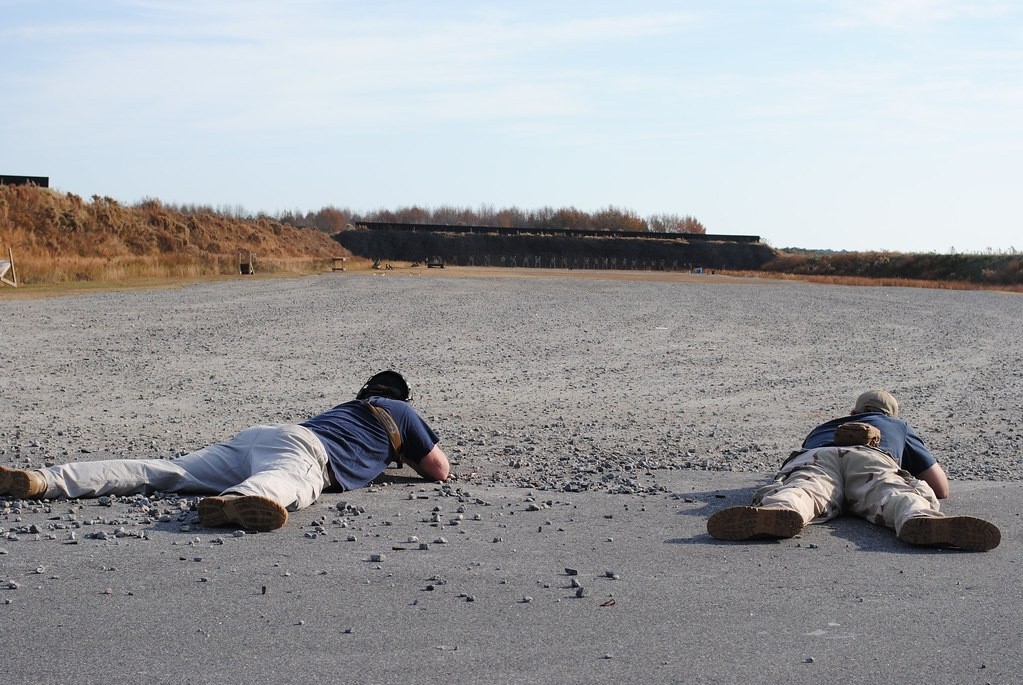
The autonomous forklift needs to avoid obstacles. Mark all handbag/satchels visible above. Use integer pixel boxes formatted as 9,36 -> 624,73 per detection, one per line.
834,421 -> 881,446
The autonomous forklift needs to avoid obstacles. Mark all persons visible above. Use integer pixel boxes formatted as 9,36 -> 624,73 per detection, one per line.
0,370 -> 450,533
707,390 -> 1001,551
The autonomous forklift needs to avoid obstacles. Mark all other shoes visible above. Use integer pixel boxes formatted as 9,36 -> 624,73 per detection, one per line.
198,492 -> 288,531
0,466 -> 48,499
900,515 -> 1001,551
707,506 -> 804,541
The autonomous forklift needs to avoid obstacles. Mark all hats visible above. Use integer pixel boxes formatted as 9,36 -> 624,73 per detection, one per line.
366,371 -> 410,396
855,389 -> 898,418
